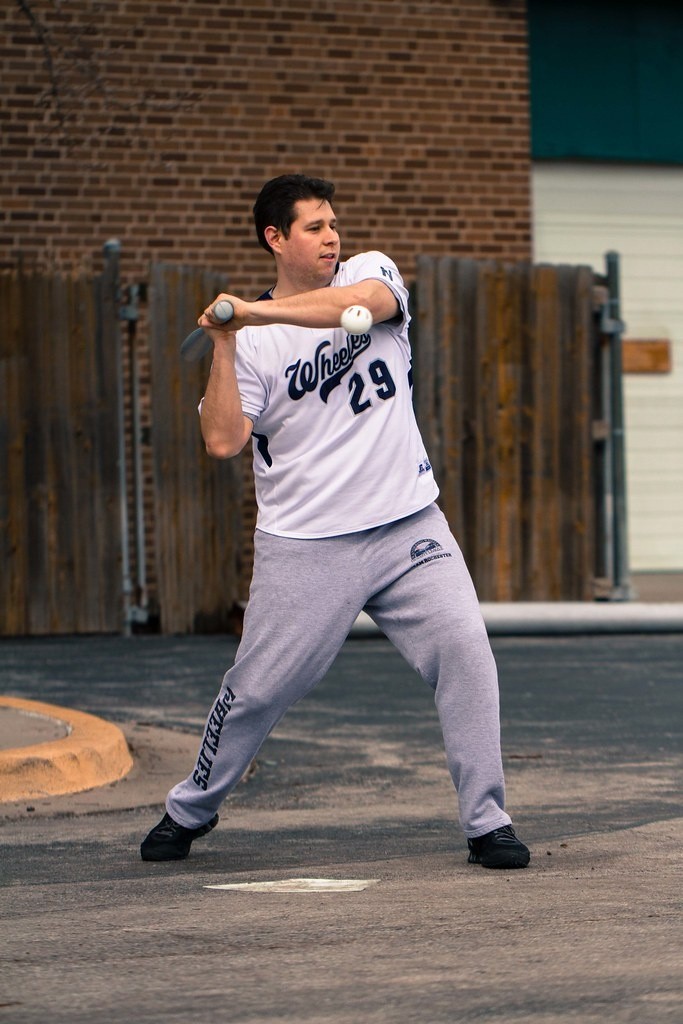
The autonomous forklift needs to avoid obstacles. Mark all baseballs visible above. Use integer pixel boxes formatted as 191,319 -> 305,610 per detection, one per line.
340,305 -> 374,336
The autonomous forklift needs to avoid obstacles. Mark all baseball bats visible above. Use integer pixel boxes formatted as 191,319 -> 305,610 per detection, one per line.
179,299 -> 234,364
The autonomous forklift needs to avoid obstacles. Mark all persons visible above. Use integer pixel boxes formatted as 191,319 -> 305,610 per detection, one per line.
141,173 -> 531,868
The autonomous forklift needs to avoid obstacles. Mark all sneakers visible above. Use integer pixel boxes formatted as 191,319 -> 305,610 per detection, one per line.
140,811 -> 221,862
467,822 -> 530,869
